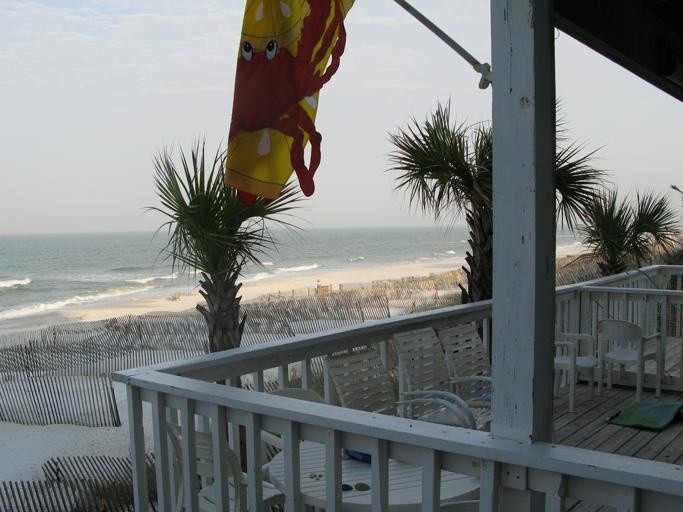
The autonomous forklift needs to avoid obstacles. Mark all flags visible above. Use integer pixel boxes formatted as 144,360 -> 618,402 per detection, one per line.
219,1 -> 353,209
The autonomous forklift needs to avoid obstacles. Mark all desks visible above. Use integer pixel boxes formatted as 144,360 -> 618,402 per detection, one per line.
268,440 -> 481,511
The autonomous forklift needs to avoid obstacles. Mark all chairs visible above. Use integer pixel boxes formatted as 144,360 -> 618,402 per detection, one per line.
257,388 -> 328,480
598,319 -> 664,403
167,421 -> 285,512
554,320 -> 595,413
323,347 -> 469,429
391,325 -> 491,431
